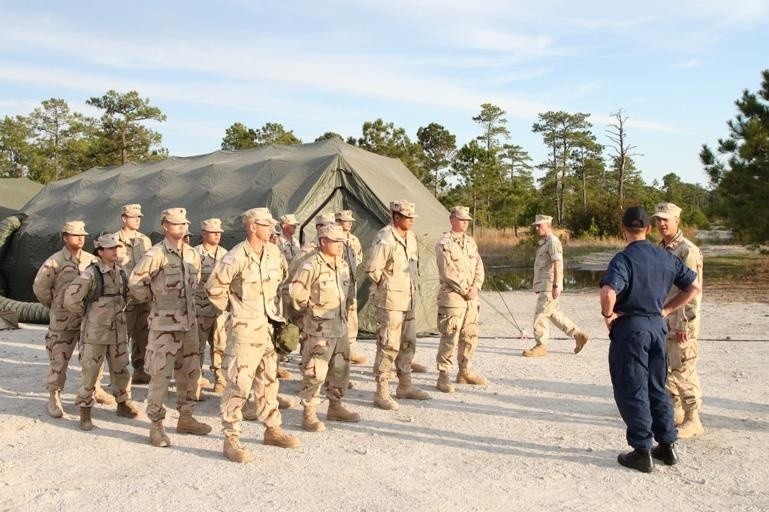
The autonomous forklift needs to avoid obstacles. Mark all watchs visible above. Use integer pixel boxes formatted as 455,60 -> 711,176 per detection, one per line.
601,311 -> 614,318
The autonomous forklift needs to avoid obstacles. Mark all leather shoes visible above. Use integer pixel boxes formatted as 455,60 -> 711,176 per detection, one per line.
618,452 -> 653,473
652,444 -> 678,465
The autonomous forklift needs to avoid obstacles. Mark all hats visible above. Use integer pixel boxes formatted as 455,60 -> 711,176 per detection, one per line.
335,210 -> 356,221
241,208 -> 280,226
531,214 -> 553,226
651,203 -> 682,219
161,208 -> 191,225
121,204 -> 144,217
62,221 -> 89,236
94,233 -> 122,249
201,218 -> 224,233
450,206 -> 473,220
316,213 -> 340,226
280,214 -> 300,226
317,224 -> 350,241
623,205 -> 650,227
394,204 -> 419,218
391,199 -> 408,209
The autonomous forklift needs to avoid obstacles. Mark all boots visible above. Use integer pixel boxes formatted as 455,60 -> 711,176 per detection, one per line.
200,378 -> 209,386
133,371 -> 151,382
277,395 -> 291,408
117,404 -> 138,418
214,381 -> 227,392
223,436 -> 246,461
242,402 -> 257,420
674,411 -> 704,439
522,346 -> 545,357
80,412 -> 92,430
48,396 -> 63,417
438,376 -> 455,393
177,418 -> 212,434
574,332 -> 588,354
264,428 -> 300,448
396,381 -> 430,400
278,368 -> 291,377
151,422 -> 170,447
673,407 -> 684,426
353,355 -> 367,363
348,382 -> 352,388
303,407 -> 326,433
457,370 -> 484,385
327,403 -> 360,422
374,392 -> 400,411
94,390 -> 115,404
411,361 -> 426,372
186,391 -> 204,400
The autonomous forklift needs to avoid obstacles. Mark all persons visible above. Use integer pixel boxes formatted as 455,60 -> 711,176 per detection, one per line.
32,220 -> 113,419
287,224 -> 361,432
129,208 -> 212,447
192,219 -> 228,391
268,230 -> 290,379
64,233 -> 137,430
367,202 -> 432,410
336,208 -> 367,365
203,208 -> 297,462
240,205 -> 290,421
521,215 -> 591,357
434,206 -> 484,392
369,199 -> 429,371
653,202 -> 702,439
600,205 -> 701,474
112,202 -> 151,382
288,212 -> 353,389
279,214 -> 301,263
